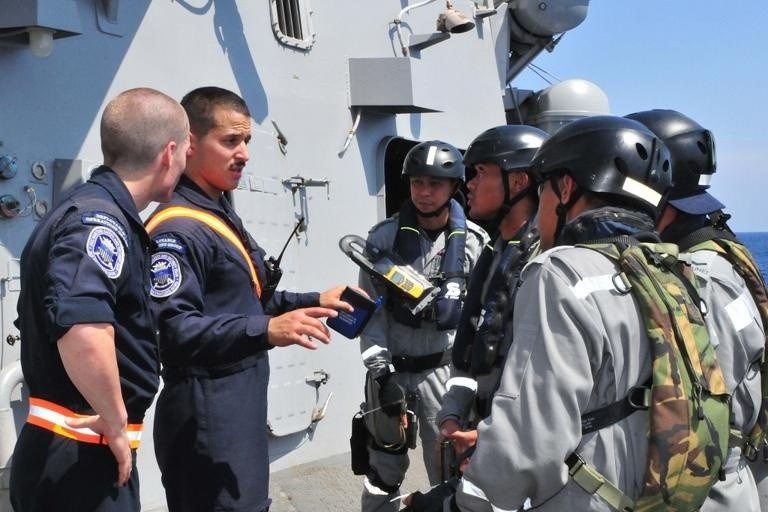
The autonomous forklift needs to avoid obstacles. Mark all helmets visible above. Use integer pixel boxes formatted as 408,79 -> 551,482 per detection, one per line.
463,109 -> 717,226
403,140 -> 465,182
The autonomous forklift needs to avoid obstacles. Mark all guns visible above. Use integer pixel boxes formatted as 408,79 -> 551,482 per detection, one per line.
401,389 -> 422,449
440,439 -> 456,480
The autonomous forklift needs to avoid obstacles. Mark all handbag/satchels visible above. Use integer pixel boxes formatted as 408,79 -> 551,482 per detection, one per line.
618,244 -> 733,512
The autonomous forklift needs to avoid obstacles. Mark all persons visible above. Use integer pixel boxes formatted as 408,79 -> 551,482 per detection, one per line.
626,107 -> 768,511
399,116 -> 719,512
144,85 -> 376,512
436,123 -> 554,512
8,87 -> 191,512
352,139 -> 492,512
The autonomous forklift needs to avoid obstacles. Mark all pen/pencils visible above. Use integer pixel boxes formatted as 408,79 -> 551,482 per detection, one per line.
356,296 -> 384,339
